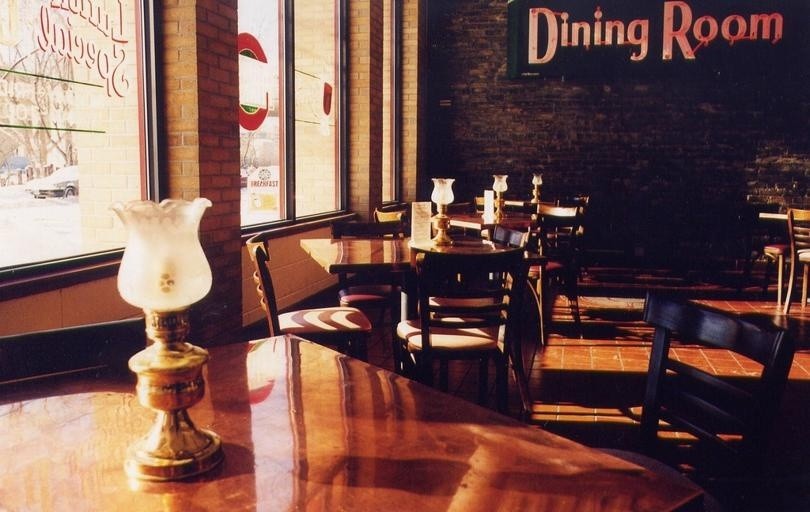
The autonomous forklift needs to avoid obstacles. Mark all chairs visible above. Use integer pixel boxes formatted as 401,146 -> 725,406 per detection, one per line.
0,195 -> 810,512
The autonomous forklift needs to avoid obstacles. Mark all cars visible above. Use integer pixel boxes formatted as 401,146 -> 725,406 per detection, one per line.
25,166 -> 79,199
240,164 -> 259,189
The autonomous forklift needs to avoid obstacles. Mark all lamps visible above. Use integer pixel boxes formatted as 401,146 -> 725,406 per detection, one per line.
433,173 -> 544,245
